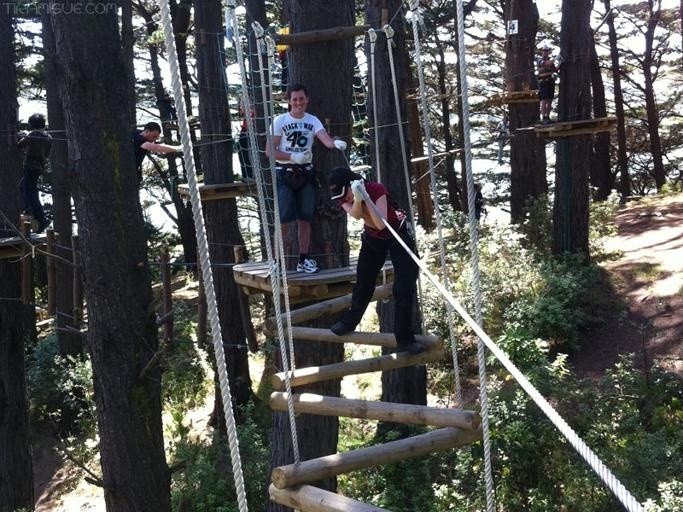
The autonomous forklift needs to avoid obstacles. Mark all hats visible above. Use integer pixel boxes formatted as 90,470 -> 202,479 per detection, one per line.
540,44 -> 552,52
328,167 -> 352,201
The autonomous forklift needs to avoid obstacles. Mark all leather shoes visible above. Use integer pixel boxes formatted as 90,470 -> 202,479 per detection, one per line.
331,314 -> 356,336
396,337 -> 426,355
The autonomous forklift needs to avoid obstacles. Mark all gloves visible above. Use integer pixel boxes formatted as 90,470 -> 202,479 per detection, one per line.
290,151 -> 310,164
333,139 -> 347,152
350,179 -> 369,202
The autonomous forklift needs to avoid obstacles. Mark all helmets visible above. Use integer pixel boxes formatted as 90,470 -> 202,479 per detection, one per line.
29,114 -> 45,129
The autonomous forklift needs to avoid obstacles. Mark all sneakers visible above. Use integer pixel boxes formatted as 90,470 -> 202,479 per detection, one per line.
542,117 -> 553,125
297,258 -> 321,273
36,217 -> 51,234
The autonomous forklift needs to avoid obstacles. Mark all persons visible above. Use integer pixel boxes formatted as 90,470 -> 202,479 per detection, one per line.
535,44 -> 558,128
263,82 -> 349,276
326,165 -> 425,357
14,114 -> 53,232
474,183 -> 484,222
237,96 -> 256,184
134,121 -> 183,169
